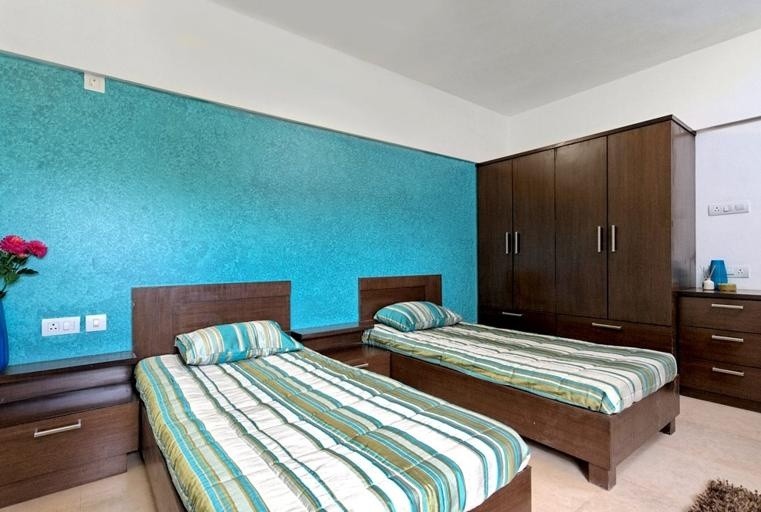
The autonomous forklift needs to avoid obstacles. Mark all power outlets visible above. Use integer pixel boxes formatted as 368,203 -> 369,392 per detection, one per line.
709,201 -> 724,216
41,318 -> 61,337
737,265 -> 750,278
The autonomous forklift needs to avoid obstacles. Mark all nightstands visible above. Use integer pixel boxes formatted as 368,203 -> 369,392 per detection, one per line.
0,350 -> 140,506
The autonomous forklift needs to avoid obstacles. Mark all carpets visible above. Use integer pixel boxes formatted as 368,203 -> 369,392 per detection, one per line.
687,477 -> 761,512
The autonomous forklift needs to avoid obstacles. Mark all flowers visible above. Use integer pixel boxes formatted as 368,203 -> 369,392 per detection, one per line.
1,235 -> 48,299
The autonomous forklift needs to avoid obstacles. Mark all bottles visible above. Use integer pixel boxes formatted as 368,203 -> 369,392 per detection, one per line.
717,283 -> 738,292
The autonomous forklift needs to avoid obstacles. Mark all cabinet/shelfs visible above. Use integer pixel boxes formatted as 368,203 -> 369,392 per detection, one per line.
475,115 -> 697,376
674,286 -> 761,412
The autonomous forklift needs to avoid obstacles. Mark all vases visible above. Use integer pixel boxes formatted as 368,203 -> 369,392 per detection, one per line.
0,301 -> 10,376
